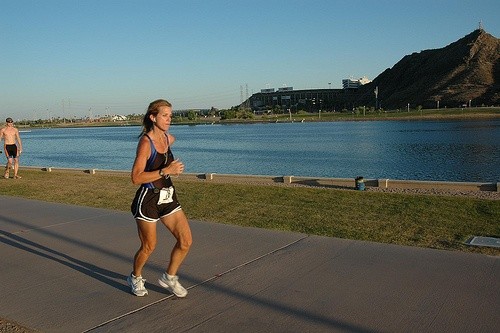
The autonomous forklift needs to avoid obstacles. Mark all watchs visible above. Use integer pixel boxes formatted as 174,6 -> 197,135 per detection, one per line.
159,169 -> 165,178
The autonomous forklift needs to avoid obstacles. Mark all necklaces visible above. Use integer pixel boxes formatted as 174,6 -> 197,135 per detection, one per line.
151,130 -> 167,164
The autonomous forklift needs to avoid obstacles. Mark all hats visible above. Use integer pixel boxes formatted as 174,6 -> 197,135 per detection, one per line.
6,117 -> 13,121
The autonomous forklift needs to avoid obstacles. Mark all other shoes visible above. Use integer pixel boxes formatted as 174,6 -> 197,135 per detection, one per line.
13,174 -> 22,179
3,171 -> 9,178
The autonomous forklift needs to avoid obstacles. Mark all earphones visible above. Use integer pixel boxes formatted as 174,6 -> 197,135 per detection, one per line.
153,118 -> 156,123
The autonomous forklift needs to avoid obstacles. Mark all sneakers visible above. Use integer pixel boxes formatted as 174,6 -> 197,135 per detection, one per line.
159,271 -> 188,298
125,271 -> 148,297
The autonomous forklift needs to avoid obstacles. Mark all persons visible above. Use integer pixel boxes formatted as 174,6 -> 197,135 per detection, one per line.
127,99 -> 192,298
0,118 -> 22,180
